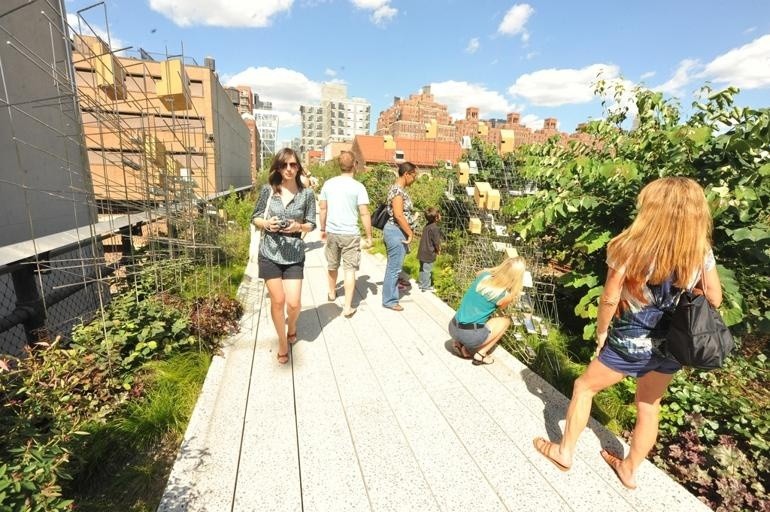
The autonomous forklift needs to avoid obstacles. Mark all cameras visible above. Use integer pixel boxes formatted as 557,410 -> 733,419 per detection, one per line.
274,220 -> 292,233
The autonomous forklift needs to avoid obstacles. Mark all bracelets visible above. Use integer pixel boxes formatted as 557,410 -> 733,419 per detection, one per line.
320,229 -> 325,232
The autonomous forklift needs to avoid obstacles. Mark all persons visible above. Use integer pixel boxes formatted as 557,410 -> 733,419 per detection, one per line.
249,147 -> 317,365
416,206 -> 442,292
532,176 -> 723,489
381,161 -> 418,311
317,150 -> 373,319
448,255 -> 527,366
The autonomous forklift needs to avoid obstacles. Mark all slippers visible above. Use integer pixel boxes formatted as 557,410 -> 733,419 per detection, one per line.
534,437 -> 570,472
278,334 -> 296,366
601,449 -> 637,489
344,310 -> 356,318
328,292 -> 336,301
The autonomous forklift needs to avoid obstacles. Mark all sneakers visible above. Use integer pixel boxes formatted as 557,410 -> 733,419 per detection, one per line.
384,304 -> 404,310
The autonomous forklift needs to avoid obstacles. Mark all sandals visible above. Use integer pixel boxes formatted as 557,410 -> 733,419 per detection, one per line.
455,342 -> 494,365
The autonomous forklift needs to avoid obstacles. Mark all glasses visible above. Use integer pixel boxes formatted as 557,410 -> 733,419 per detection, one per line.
280,163 -> 297,168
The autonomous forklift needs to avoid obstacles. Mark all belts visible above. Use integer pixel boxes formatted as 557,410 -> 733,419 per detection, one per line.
452,317 -> 484,329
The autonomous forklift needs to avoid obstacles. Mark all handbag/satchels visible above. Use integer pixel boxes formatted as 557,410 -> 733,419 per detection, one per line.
667,292 -> 732,369
371,202 -> 389,230
